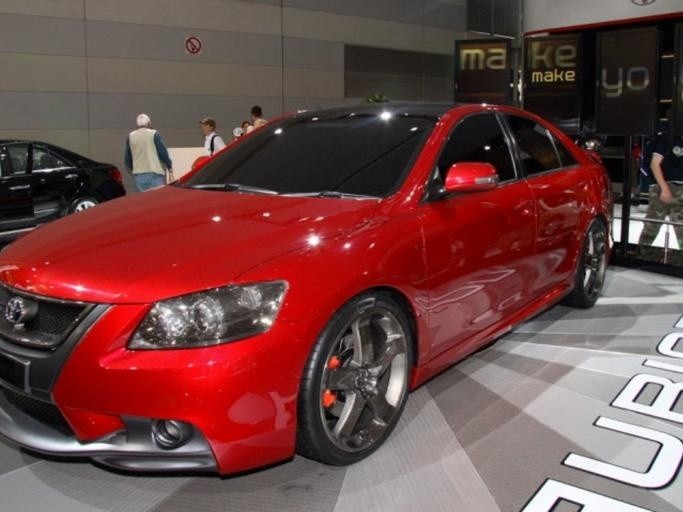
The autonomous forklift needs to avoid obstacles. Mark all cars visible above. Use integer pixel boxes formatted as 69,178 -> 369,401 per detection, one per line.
0,140 -> 125,242
0,103 -> 615,476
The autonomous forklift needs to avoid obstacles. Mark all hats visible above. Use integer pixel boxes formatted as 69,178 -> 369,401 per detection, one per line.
200,118 -> 215,127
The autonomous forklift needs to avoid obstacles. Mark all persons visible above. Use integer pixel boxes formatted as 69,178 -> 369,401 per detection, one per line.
125,113 -> 175,191
631,141 -> 641,189
232,105 -> 267,138
639,131 -> 683,252
199,117 -> 226,156
